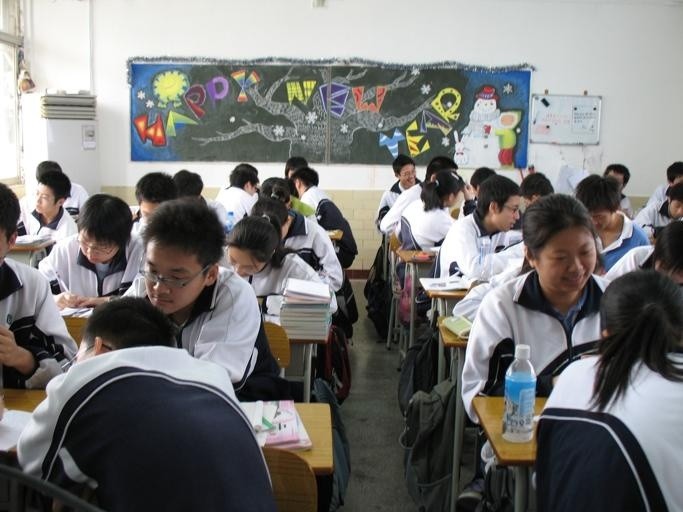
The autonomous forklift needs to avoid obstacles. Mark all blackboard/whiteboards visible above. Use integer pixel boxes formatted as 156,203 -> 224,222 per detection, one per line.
529,94 -> 603,145
131,64 -> 531,169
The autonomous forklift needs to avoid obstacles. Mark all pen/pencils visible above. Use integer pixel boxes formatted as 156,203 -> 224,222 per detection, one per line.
61,280 -> 69,293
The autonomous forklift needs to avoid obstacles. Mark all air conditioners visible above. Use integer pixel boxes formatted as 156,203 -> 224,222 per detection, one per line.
19,89 -> 98,203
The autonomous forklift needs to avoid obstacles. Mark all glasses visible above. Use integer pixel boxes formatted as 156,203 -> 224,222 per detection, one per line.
78,235 -> 115,255
138,253 -> 209,288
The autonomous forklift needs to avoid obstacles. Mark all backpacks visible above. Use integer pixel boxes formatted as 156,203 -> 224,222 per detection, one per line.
364,245 -> 514,512
312,270 -> 358,512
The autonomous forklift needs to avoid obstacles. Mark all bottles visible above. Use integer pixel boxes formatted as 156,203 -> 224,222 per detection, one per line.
501,344 -> 538,442
477,238 -> 495,282
223,212 -> 237,230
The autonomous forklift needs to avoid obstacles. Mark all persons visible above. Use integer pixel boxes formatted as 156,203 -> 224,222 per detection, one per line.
453,242 -> 606,500
15,293 -> 281,512
1,155 -> 682,380
532,269 -> 683,511
0,182 -> 79,389
120,194 -> 281,402
461,193 -> 609,481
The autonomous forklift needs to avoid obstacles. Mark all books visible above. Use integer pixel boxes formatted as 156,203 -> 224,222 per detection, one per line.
243,398 -> 313,453
